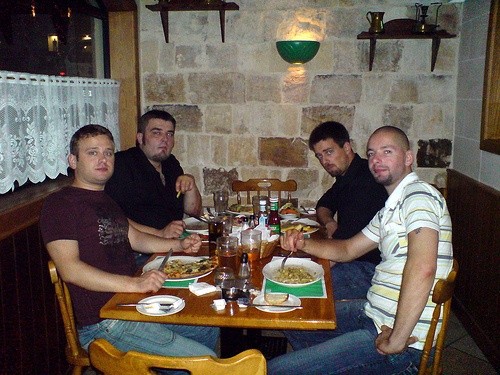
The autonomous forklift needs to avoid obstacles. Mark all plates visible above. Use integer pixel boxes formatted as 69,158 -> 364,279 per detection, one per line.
142,256 -> 214,282
278,211 -> 299,220
298,206 -> 316,214
181,216 -> 210,235
253,294 -> 301,313
281,218 -> 322,235
225,205 -> 255,216
261,257 -> 324,288
135,294 -> 186,316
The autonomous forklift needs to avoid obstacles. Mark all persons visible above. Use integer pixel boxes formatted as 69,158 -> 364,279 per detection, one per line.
39,124 -> 219,375
267,126 -> 453,375
309,121 -> 389,299
104,108 -> 202,267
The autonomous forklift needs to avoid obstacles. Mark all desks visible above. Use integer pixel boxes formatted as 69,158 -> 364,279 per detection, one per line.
99,208 -> 336,356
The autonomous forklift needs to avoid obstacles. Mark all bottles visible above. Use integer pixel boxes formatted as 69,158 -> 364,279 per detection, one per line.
213,195 -> 282,288
241,229 -> 261,263
217,237 -> 239,273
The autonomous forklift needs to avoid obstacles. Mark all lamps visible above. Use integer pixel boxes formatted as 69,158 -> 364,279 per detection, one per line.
277,39 -> 320,65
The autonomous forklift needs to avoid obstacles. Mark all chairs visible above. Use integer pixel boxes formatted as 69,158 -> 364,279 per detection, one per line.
48,258 -> 91,375
418,259 -> 459,374
86,338 -> 266,375
231,178 -> 296,213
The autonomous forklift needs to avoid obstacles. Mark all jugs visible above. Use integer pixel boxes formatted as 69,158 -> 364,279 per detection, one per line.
366,10 -> 385,34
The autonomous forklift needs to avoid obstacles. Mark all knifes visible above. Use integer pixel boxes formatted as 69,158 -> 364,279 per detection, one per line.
148,248 -> 174,293
242,303 -> 303,308
